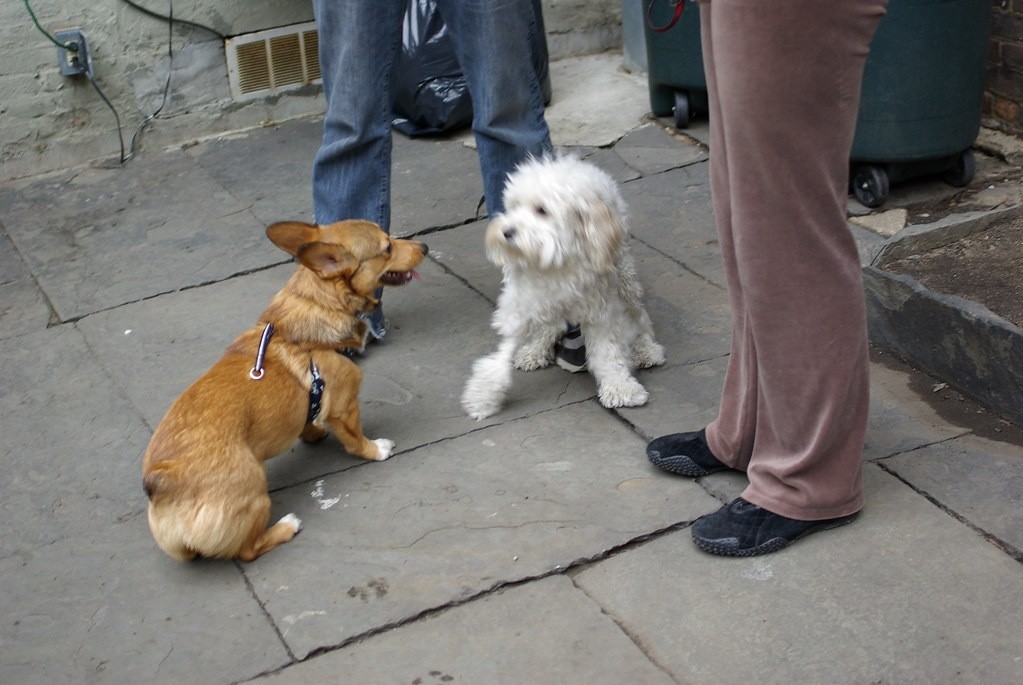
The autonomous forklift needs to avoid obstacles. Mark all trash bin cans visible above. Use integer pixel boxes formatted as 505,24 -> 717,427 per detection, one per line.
848,0 -> 992,208
638,0 -> 710,129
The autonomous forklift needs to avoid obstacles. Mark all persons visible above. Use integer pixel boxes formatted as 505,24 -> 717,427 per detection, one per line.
646,0 -> 889,559
309,0 -> 588,372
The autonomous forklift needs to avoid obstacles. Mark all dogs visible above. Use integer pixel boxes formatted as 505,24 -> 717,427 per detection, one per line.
458,148 -> 666,423
141,218 -> 429,563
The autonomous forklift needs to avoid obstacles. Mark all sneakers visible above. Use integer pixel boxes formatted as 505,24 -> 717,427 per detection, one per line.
691,496 -> 859,557
646,428 -> 737,476
555,324 -> 587,373
